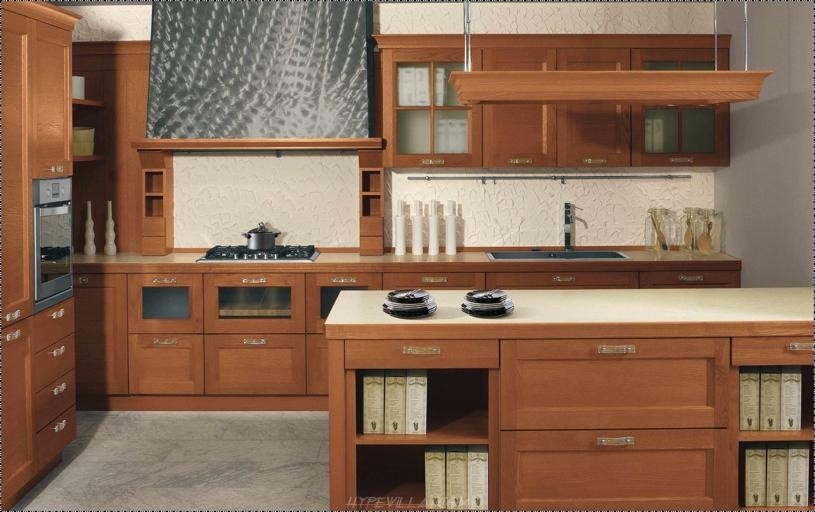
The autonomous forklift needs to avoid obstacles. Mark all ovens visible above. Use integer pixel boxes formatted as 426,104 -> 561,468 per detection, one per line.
31,174 -> 75,313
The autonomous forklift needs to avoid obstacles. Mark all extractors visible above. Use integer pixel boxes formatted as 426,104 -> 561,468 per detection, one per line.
135,1 -> 387,152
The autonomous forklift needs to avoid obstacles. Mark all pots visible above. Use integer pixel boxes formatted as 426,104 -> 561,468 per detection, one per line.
243,224 -> 281,251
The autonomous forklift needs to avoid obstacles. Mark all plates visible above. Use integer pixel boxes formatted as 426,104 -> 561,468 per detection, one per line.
382,303 -> 438,319
461,303 -> 515,318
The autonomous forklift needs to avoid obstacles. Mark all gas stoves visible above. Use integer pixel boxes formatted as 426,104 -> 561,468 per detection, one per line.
205,245 -> 315,262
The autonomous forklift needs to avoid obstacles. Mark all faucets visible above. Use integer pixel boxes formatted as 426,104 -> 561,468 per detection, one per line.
572,205 -> 588,246
564,202 -> 574,245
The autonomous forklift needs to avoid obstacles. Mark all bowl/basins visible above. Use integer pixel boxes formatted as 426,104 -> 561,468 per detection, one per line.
465,290 -> 508,310
387,289 -> 429,308
72,125 -> 95,156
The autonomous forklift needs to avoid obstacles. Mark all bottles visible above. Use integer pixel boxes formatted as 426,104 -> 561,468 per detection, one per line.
645,208 -> 725,254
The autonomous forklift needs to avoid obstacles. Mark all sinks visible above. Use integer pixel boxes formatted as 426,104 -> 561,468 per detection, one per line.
486,246 -> 629,260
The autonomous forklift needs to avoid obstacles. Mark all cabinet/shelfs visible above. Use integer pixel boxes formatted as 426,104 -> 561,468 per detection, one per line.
2,1 -> 79,507
207,266 -> 382,412
368,32 -> 482,169
321,284 -> 815,512
482,34 -> 635,170
629,32 -> 735,170
75,263 -> 205,413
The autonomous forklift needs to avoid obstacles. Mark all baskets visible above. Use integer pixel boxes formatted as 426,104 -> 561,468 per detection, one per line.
72,126 -> 94,156
